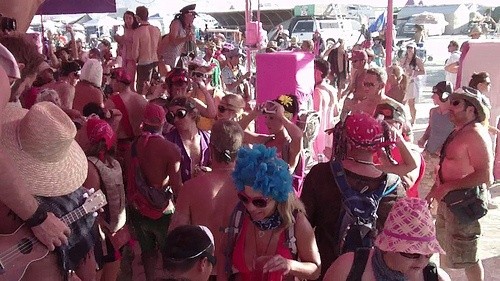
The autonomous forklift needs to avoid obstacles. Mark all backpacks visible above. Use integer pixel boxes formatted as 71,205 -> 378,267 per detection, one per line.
89,157 -> 126,233
328,157 -> 401,252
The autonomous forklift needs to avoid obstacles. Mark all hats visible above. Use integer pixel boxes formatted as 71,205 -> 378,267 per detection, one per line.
179,4 -> 198,15
0,100 -> 89,197
450,85 -> 487,122
268,41 -> 278,48
373,196 -> 448,255
291,36 -> 298,44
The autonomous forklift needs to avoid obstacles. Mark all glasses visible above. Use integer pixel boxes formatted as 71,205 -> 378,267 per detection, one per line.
452,99 -> 461,107
238,190 -> 270,209
257,99 -> 276,114
203,251 -> 217,267
166,108 -> 188,121
364,82 -> 380,88
218,106 -> 233,113
401,253 -> 434,258
354,59 -> 362,64
190,69 -> 213,79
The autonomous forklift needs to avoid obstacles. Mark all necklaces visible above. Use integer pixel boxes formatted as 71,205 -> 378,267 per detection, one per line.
344,156 -> 375,165
254,224 -> 274,257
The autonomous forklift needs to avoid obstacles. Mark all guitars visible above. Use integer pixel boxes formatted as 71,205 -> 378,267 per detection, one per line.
0,187 -> 107,281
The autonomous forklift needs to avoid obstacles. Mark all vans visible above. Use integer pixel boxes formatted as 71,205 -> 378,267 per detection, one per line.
291,19 -> 366,51
404,12 -> 446,36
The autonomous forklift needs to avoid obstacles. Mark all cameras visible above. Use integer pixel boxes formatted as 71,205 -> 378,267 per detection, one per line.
0,15 -> 16,32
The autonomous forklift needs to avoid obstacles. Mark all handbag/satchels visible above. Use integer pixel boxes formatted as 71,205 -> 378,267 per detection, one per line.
446,186 -> 488,221
127,150 -> 172,220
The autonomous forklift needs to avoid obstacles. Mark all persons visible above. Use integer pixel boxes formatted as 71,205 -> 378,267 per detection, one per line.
425,85 -> 493,281
0,0 -> 500,281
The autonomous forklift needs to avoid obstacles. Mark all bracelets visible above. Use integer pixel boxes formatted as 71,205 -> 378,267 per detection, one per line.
25,206 -> 48,228
